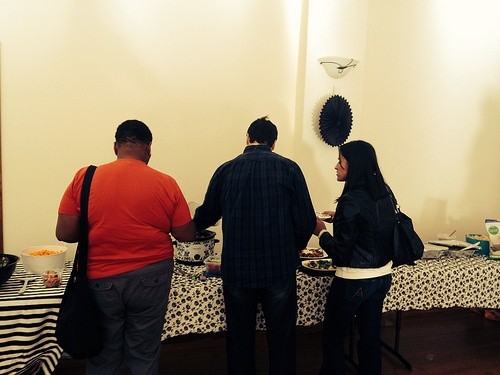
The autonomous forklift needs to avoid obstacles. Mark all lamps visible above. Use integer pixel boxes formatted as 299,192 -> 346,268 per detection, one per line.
319,56 -> 359,79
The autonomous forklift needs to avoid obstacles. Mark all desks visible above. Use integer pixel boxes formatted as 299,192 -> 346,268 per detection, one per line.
0,243 -> 500,375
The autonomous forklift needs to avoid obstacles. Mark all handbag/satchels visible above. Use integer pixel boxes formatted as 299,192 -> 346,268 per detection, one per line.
55,257 -> 103,361
383,180 -> 426,268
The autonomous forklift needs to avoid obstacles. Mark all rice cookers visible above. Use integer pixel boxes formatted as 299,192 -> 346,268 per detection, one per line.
172,230 -> 220,266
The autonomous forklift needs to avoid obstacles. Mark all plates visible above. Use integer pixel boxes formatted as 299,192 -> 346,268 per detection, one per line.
315,211 -> 331,220
302,258 -> 336,271
300,247 -> 328,259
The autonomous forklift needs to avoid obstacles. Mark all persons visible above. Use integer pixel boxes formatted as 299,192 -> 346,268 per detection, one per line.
55,120 -> 196,375
313,140 -> 397,375
193,116 -> 317,375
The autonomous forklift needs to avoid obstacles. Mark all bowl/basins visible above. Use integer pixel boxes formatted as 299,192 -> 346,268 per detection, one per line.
203,252 -> 221,272
465,234 -> 490,255
0,253 -> 20,287
18,244 -> 68,275
42,268 -> 63,287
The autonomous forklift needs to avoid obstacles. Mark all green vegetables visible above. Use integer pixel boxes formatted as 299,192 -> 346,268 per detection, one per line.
308,260 -> 336,269
